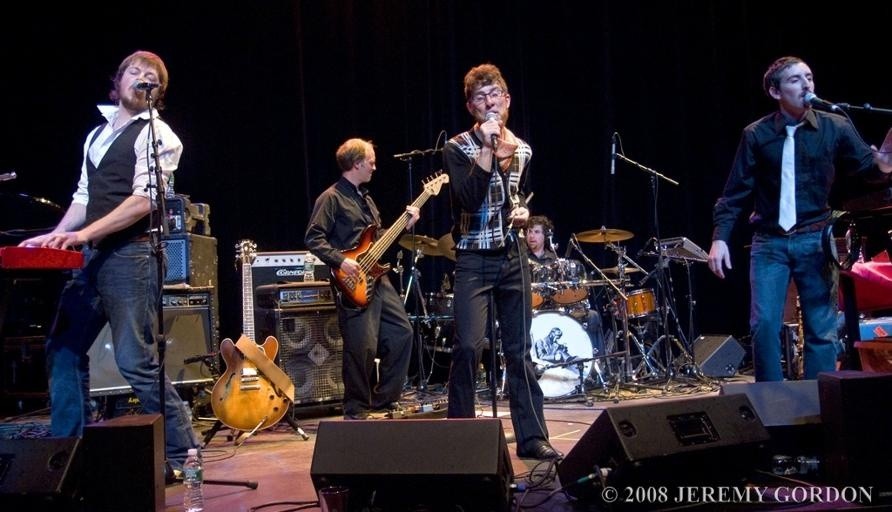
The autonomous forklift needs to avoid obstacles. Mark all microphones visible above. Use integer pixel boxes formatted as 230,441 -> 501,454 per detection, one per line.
803,93 -> 840,113
610,136 -> 617,176
394,149 -> 432,159
535,366 -> 546,381
129,77 -> 162,97
178,351 -> 217,366
0,170 -> 19,187
484,109 -> 502,152
565,237 -> 573,260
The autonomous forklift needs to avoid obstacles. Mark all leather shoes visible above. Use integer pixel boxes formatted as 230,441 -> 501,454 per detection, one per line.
517,443 -> 558,458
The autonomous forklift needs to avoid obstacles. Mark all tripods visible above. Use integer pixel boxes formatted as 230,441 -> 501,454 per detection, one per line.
522,348 -> 631,410
131,87 -> 260,497
612,147 -> 710,395
387,160 -> 461,411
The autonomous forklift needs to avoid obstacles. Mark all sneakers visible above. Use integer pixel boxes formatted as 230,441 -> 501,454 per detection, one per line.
381,401 -> 402,412
344,412 -> 374,420
173,466 -> 202,481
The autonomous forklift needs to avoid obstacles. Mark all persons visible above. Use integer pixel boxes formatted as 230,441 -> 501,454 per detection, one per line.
524,215 -> 612,388
441,64 -> 566,460
16,49 -> 205,485
303,136 -> 423,421
706,54 -> 892,383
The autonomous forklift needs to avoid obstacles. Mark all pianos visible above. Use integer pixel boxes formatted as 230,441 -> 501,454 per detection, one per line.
1,245 -> 85,275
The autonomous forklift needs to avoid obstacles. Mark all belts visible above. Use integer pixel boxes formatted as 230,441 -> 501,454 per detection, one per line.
773,221 -> 826,236
93,234 -> 151,245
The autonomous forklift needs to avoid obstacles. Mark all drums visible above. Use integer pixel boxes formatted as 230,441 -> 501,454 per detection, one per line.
530,263 -> 551,309
613,288 -> 657,318
550,259 -> 590,304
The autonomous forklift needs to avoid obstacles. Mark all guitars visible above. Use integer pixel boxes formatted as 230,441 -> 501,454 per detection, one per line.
329,169 -> 451,315
211,239 -> 294,433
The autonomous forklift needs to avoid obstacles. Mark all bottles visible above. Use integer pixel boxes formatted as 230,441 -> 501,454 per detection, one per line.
181,448 -> 205,511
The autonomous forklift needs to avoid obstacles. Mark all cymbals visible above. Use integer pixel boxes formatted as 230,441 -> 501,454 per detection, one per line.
600,267 -> 640,273
576,227 -> 634,244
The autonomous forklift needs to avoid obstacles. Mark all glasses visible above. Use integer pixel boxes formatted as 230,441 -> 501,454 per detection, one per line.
470,88 -> 505,102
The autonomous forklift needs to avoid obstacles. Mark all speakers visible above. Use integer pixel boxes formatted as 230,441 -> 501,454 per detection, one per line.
254,281 -> 362,421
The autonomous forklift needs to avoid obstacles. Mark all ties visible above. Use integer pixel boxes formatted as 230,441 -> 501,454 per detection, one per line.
778,121 -> 805,232
